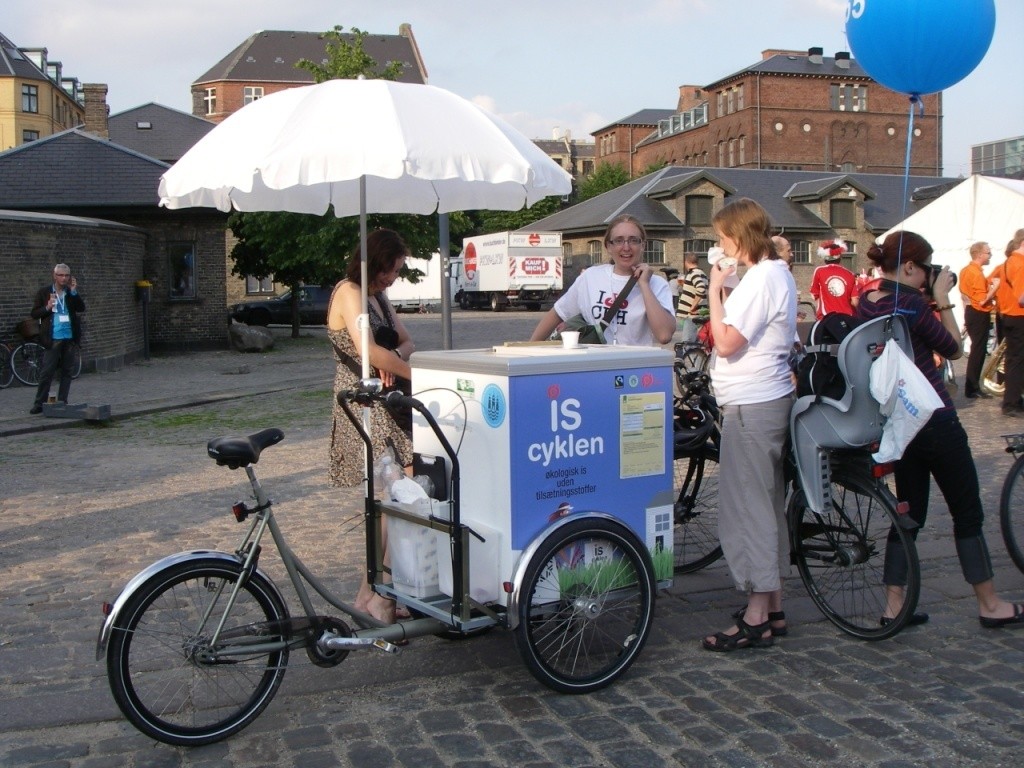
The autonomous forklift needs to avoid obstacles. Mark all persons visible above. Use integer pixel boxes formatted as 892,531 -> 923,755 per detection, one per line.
30,264 -> 86,413
530,215 -> 677,346
859,230 -> 1024,629
810,240 -> 861,317
702,197 -> 799,652
327,229 -> 416,646
959,242 -> 1000,399
999,228 -> 1024,418
770,236 -> 800,343
987,240 -> 1014,384
678,252 -> 711,353
856,268 -> 880,290
669,274 -> 683,310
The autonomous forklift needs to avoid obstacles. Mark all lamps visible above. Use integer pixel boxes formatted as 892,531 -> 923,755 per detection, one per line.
840,187 -> 857,196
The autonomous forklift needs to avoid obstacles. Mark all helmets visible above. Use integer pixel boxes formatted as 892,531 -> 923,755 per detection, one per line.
671,408 -> 715,457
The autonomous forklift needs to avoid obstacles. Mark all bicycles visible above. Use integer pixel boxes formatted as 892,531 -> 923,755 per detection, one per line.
999,434 -> 1024,578
1,319 -> 83,389
672,312 -> 921,640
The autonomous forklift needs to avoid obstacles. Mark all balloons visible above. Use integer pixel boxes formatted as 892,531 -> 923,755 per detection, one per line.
844,0 -> 996,102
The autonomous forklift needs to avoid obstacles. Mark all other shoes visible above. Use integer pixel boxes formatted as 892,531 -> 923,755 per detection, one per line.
880,611 -> 930,627
967,389 -> 994,399
1005,406 -> 1024,420
980,602 -> 1024,627
29,404 -> 42,414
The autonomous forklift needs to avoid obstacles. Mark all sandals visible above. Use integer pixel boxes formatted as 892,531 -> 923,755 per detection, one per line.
737,610 -> 787,636
702,617 -> 773,651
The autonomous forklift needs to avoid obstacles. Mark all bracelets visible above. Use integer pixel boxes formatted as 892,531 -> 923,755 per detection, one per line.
394,349 -> 402,358
937,305 -> 955,312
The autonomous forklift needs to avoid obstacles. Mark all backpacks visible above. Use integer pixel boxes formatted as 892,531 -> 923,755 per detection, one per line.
796,312 -> 873,401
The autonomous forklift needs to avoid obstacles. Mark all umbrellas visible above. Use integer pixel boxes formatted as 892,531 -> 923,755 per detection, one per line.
157,74 -> 575,498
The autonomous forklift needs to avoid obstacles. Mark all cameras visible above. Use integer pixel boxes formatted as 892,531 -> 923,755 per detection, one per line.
921,264 -> 957,295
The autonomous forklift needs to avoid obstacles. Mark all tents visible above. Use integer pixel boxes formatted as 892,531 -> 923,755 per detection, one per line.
874,174 -> 1024,354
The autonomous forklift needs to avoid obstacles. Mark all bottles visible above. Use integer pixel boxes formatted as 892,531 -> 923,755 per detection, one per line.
379,456 -> 402,500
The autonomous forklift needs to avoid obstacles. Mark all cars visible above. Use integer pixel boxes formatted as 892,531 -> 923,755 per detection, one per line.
228,286 -> 332,327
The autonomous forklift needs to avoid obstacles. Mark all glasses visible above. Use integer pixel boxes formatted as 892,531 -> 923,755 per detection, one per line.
608,237 -> 643,246
979,251 -> 991,255
55,273 -> 71,277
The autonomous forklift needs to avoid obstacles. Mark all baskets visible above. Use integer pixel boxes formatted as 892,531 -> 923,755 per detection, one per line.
17,319 -> 39,339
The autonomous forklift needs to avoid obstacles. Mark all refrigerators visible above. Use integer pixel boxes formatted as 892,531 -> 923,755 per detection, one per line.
409,344 -> 676,606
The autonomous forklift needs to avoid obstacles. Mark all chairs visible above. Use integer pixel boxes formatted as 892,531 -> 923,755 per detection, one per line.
791,314 -> 914,513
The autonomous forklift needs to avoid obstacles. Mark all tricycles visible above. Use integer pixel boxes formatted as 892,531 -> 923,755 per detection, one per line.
94,379 -> 657,746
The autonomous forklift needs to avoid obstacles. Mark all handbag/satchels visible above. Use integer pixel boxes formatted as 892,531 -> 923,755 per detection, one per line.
550,313 -> 603,344
379,374 -> 412,431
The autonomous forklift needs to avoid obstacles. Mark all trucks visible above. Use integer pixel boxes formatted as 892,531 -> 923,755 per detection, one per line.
450,231 -> 564,311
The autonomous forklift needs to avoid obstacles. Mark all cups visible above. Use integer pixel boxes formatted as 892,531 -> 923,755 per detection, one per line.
560,331 -> 580,346
718,257 -> 738,276
50,294 -> 57,305
678,273 -> 685,285
